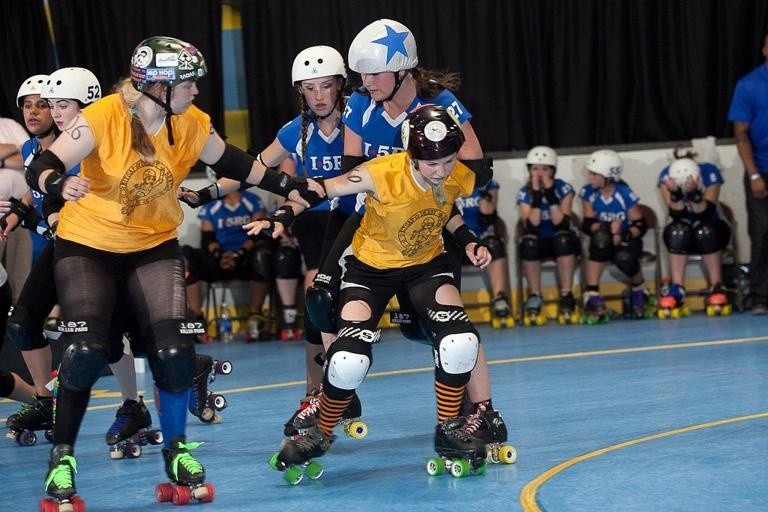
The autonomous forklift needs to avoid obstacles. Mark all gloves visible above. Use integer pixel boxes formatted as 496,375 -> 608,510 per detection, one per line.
211,247 -> 248,270
544,180 -> 560,205
0,196 -> 34,232
531,186 -> 542,208
44,169 -> 78,201
668,187 -> 684,202
621,231 -> 632,242
257,167 -> 309,199
686,186 -> 702,203
177,181 -> 221,209
601,221 -> 611,233
257,205 -> 294,232
453,224 -> 493,255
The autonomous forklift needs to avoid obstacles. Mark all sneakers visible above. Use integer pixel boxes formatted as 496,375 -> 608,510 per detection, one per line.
752,303 -> 768,315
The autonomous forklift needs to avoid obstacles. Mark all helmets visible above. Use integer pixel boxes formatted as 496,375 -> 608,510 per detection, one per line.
16,75 -> 48,108
40,67 -> 102,104
348,19 -> 419,74
291,45 -> 348,88
525,146 -> 557,168
669,157 -> 702,192
130,36 -> 209,92
586,149 -> 623,178
401,104 -> 466,160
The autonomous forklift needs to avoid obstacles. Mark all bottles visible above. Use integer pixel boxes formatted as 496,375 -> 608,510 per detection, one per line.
219,302 -> 233,345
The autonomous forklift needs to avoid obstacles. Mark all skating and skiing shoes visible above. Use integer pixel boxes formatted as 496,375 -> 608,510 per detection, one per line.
706,282 -> 732,316
188,311 -> 211,346
523,294 -> 547,326
284,388 -> 320,440
268,432 -> 336,485
40,443 -> 85,512
427,418 -> 488,477
155,435 -> 215,506
6,394 -> 55,446
188,354 -> 232,424
106,395 -> 164,459
294,382 -> 368,441
558,289 -> 579,325
630,281 -> 658,319
465,410 -> 517,464
658,284 -> 690,319
580,284 -> 609,324
492,295 -> 515,329
244,312 -> 272,343
280,305 -> 302,341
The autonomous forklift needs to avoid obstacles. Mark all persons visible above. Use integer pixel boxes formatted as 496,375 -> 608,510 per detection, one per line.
24,32 -> 327,511
657,147 -> 729,320
197,20 -> 517,483
0,68 -> 232,459
730,37 -> 767,317
577,151 -> 654,325
518,145 -> 583,327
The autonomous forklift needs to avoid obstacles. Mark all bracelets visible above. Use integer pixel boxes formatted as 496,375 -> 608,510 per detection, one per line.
750,172 -> 759,181
